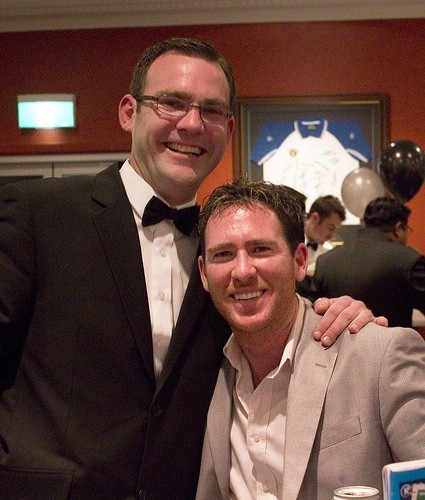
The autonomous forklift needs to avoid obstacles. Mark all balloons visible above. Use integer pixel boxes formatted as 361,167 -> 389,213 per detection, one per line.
340,168 -> 385,219
378,139 -> 425,202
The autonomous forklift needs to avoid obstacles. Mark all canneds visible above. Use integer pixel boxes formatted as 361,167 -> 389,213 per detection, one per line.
330,485 -> 380,500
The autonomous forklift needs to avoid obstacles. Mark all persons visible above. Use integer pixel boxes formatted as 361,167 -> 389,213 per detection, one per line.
296,195 -> 346,302
0,38 -> 388,500
308,197 -> 425,328
195,175 -> 425,500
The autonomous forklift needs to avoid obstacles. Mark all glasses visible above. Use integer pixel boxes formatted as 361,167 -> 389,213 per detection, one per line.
405,224 -> 413,236
135,94 -> 233,123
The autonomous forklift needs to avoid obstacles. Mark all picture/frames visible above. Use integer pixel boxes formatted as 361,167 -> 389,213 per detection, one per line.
231,94 -> 392,246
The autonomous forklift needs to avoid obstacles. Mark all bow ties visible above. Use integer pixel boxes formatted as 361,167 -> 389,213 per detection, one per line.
307,243 -> 317,251
142,196 -> 201,236
